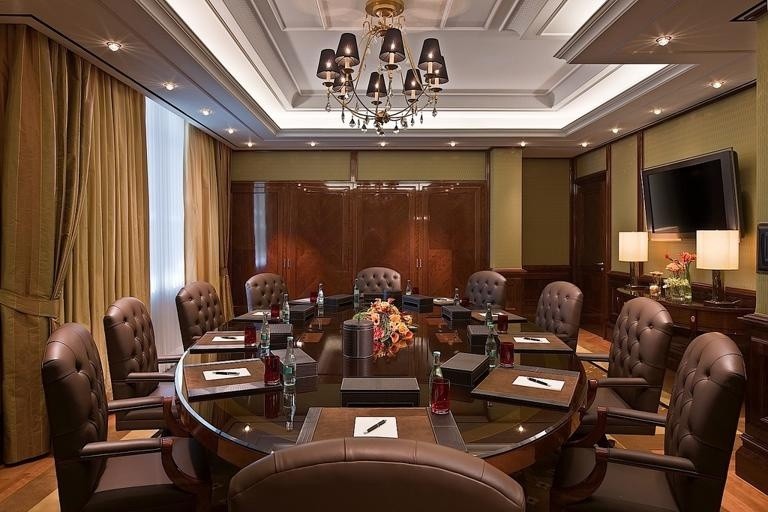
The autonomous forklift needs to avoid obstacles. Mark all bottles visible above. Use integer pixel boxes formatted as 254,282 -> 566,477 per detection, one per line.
485,324 -> 501,367
282,294 -> 291,322
354,279 -> 359,299
454,288 -> 460,305
317,308 -> 324,330
317,283 -> 324,308
429,351 -> 444,409
406,280 -> 412,296
259,348 -> 269,360
259,312 -> 271,348
486,303 -> 492,327
283,336 -> 297,386
282,386 -> 296,431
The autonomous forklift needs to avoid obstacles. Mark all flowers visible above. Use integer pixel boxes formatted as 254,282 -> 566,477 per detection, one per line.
661,250 -> 696,285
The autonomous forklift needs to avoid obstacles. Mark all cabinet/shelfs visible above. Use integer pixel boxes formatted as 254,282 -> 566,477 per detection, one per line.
733,312 -> 768,498
616,284 -> 754,376
353,180 -> 490,303
230,180 -> 353,316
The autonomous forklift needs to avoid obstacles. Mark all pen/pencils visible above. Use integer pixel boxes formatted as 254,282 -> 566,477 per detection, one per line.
524,337 -> 540,341
493,314 -> 500,315
364,419 -> 387,434
437,299 -> 447,300
212,372 -> 240,375
528,377 -> 548,385
222,337 -> 236,339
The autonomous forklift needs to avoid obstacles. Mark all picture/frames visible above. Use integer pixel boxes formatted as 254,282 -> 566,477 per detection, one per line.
756,223 -> 768,275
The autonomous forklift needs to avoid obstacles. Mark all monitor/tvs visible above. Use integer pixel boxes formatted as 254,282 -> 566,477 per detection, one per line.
639,147 -> 745,242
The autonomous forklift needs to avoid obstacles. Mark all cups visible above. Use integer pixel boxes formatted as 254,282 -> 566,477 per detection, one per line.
264,392 -> 281,420
270,304 -> 280,319
501,342 -> 514,367
244,326 -> 256,346
498,314 -> 507,331
264,355 -> 281,385
430,377 -> 452,415
310,292 -> 317,304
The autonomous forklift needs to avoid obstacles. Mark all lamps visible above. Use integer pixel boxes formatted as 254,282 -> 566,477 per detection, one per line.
694,229 -> 743,305
617,229 -> 648,289
315,0 -> 448,137
